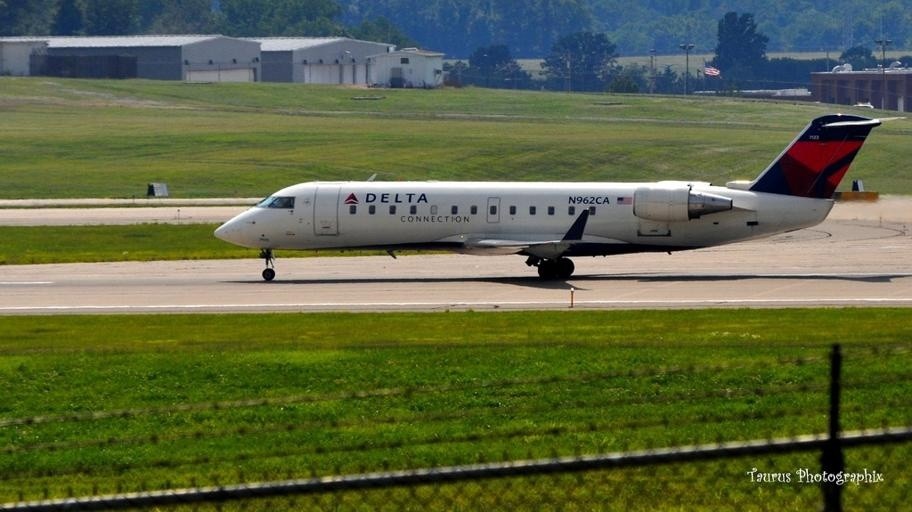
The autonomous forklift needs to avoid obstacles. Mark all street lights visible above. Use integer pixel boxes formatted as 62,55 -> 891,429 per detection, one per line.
482,54 -> 489,88
565,49 -> 571,92
678,43 -> 694,96
875,38 -> 892,111
648,49 -> 656,94
451,56 -> 458,86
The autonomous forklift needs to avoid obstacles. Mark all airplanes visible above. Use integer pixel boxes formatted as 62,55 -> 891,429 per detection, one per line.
213,110 -> 884,286
